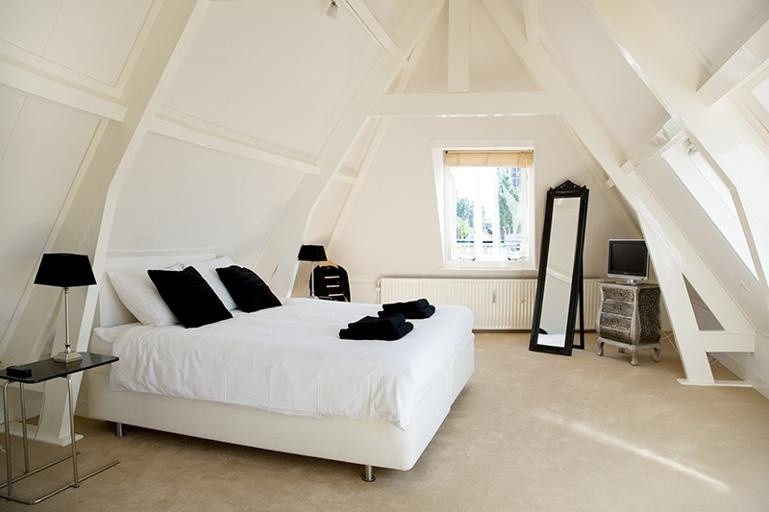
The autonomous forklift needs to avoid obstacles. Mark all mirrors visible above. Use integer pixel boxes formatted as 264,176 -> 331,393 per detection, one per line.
527,178 -> 590,357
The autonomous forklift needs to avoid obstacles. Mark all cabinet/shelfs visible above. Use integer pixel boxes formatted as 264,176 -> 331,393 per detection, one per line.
593,282 -> 663,367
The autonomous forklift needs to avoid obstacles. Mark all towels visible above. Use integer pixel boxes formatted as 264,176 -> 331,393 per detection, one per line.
336,297 -> 436,342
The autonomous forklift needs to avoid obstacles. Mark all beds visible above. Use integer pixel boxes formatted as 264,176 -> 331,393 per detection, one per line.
71,246 -> 477,483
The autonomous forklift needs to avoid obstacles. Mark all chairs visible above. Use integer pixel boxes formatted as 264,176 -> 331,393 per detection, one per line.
311,262 -> 351,302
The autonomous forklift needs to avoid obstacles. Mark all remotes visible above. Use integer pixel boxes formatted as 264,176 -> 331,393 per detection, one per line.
7,365 -> 33,375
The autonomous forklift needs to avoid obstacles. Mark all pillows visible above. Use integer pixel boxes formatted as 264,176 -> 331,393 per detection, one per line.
103,255 -> 283,330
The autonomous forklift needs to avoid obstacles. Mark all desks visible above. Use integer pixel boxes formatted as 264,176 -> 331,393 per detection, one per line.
0,351 -> 120,505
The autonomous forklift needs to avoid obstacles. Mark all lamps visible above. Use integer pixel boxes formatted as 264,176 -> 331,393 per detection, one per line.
296,244 -> 328,299
33,252 -> 99,364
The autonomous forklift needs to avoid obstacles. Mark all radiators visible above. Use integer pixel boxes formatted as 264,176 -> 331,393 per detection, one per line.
379,276 -> 605,331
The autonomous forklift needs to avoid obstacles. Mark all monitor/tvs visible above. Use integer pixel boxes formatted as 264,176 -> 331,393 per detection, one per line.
606,238 -> 650,286
